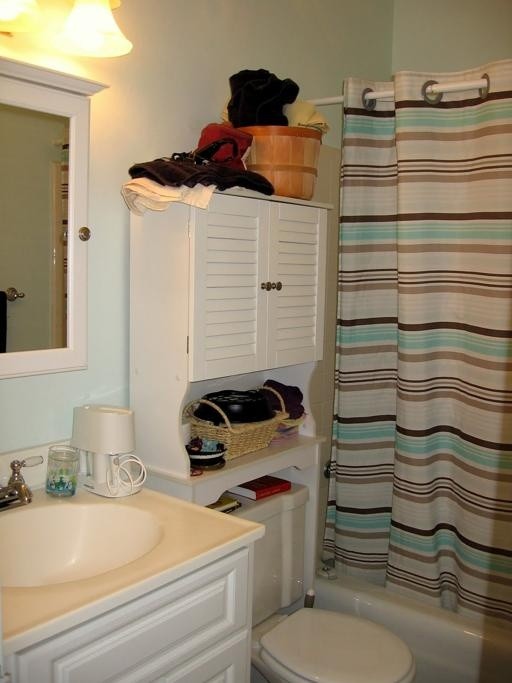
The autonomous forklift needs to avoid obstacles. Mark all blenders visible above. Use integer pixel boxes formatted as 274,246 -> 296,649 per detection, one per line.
67,404 -> 147,500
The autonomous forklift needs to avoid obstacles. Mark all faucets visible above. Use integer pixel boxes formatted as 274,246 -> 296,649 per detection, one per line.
0,457 -> 43,507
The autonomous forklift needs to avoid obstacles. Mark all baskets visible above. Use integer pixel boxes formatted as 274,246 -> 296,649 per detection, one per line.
182,386 -> 289,460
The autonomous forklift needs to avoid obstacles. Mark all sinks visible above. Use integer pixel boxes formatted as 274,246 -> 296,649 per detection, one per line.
0,503 -> 163,588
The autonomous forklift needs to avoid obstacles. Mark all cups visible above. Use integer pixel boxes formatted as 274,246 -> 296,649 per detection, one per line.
44,443 -> 82,498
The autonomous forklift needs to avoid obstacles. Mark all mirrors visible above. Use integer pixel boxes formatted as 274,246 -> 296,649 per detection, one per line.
0,55 -> 110,378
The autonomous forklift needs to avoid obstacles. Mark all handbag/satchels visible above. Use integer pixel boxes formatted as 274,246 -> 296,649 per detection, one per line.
128,136 -> 274,197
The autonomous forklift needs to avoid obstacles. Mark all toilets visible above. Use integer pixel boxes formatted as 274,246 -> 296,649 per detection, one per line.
222,483 -> 416,683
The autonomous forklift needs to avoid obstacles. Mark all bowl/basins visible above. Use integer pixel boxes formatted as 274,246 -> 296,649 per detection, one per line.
189,444 -> 227,463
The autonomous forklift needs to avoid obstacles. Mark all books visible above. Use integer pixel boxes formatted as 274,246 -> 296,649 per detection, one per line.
205,495 -> 238,511
227,474 -> 291,501
221,502 -> 241,513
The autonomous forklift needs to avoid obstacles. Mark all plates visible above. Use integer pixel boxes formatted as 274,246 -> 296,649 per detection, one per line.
190,459 -> 226,471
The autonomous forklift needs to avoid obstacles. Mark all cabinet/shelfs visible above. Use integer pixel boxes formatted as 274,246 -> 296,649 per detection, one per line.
125,184 -> 336,594
2,539 -> 252,682
